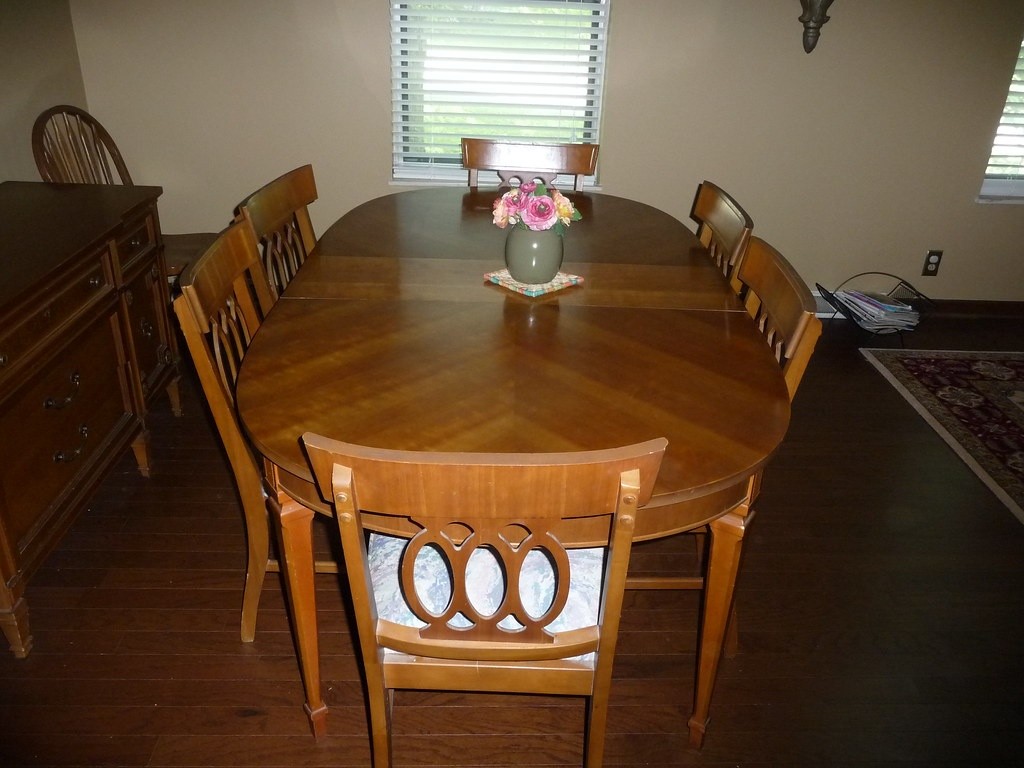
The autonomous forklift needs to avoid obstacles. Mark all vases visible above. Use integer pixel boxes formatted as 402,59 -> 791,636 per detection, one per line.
504,222 -> 564,284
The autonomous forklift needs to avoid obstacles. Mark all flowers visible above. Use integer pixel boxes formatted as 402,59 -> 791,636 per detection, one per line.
490,179 -> 582,238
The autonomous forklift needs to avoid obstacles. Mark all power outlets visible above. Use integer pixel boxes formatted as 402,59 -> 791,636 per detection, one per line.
921,251 -> 943,276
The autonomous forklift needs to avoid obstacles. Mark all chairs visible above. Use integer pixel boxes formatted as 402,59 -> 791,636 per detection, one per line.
32,104 -> 220,276
234,163 -> 318,302
461,138 -> 600,191
299,432 -> 671,768
693,180 -> 754,279
626,235 -> 823,662
172,220 -> 344,644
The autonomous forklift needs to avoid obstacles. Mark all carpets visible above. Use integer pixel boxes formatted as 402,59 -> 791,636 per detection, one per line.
858,347 -> 1024,525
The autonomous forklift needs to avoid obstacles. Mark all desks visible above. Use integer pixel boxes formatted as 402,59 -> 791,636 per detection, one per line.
234,185 -> 790,751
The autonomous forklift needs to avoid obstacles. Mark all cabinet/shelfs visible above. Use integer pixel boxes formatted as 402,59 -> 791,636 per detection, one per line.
0,181 -> 188,658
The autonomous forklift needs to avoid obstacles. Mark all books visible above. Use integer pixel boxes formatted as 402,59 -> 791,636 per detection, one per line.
834,289 -> 920,334
